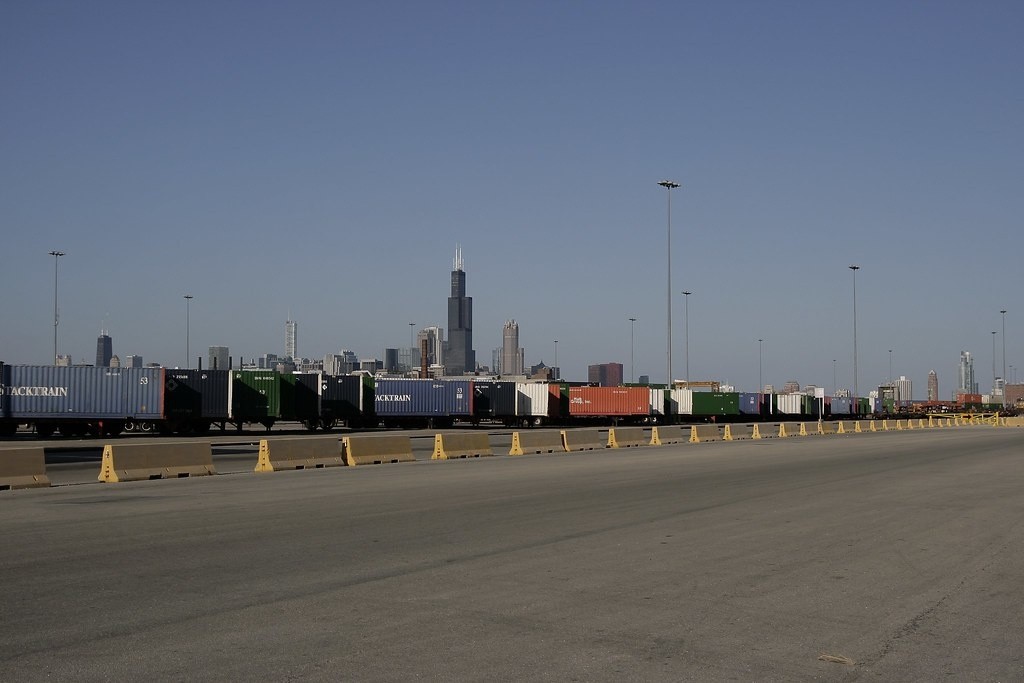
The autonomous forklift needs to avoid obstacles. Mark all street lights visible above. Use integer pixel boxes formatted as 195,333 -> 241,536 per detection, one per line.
1000,308 -> 1007,397
184,294 -> 194,370
758,339 -> 763,393
629,317 -> 637,384
409,321 -> 418,379
657,179 -> 682,389
833,359 -> 837,397
48,248 -> 67,365
992,331 -> 996,395
889,349 -> 892,399
848,263 -> 860,397
553,340 -> 559,381
682,290 -> 693,388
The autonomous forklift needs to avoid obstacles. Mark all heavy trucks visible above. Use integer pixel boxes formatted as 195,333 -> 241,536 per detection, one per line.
1,361 -> 1023,439
